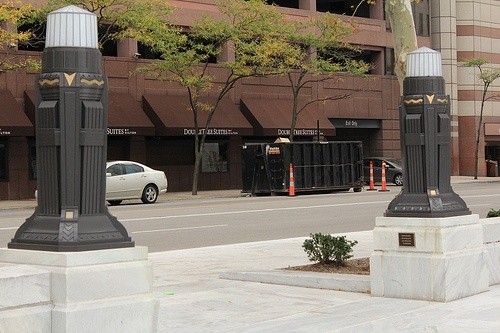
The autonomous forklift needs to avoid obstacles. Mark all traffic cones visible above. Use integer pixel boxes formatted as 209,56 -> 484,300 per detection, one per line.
378,160 -> 390,192
289,163 -> 297,197
366,160 -> 377,191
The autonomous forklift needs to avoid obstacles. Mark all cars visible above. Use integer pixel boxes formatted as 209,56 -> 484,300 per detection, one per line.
362,157 -> 403,186
35,160 -> 168,205
